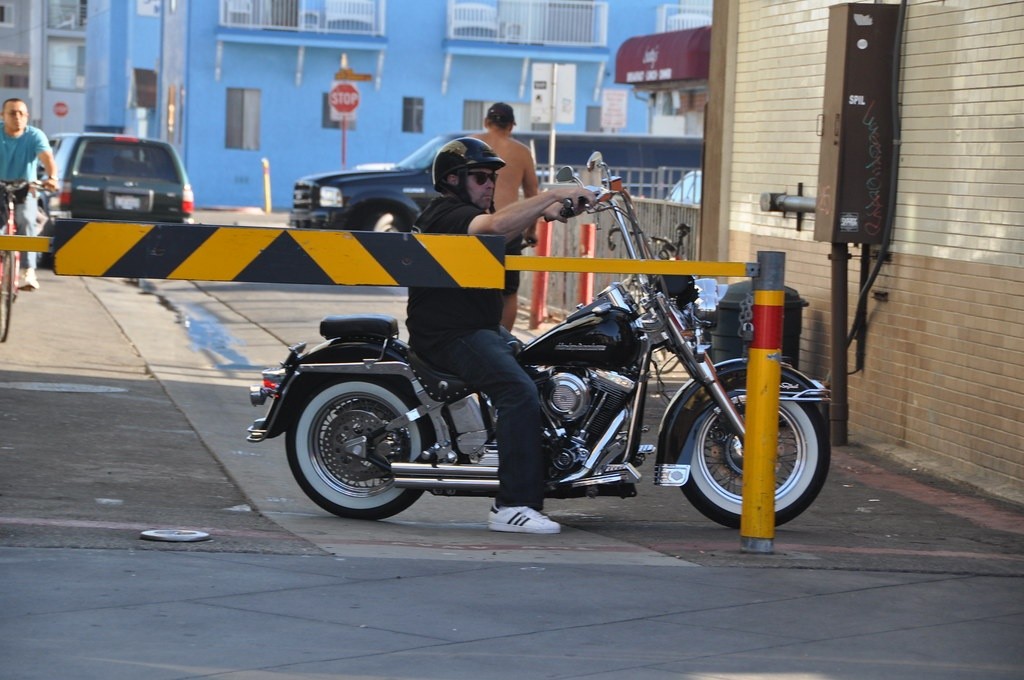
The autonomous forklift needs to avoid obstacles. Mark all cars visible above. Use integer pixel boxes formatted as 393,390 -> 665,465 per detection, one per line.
290,131 -> 706,233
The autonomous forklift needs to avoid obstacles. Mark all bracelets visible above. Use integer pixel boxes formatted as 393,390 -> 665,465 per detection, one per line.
49,176 -> 57,180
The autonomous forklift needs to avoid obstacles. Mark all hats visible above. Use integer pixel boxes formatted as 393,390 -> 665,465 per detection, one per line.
487,102 -> 516,125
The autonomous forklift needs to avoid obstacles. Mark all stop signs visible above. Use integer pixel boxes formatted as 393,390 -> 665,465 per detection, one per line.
331,82 -> 360,115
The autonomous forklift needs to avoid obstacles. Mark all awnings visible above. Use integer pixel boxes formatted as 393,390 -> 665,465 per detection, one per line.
615,25 -> 711,89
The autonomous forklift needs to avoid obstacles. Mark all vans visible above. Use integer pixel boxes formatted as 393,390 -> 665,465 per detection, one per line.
33,132 -> 196,231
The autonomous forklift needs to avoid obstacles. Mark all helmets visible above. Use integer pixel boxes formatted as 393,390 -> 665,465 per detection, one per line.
432,137 -> 505,192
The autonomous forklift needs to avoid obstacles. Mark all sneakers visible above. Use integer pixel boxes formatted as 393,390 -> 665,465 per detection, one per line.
22,268 -> 39,290
488,504 -> 560,534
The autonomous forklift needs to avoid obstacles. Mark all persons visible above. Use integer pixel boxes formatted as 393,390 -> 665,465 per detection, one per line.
405,136 -> 597,534
473,103 -> 539,330
0,98 -> 58,292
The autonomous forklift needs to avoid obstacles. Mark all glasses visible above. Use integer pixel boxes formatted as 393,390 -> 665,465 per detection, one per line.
457,172 -> 497,185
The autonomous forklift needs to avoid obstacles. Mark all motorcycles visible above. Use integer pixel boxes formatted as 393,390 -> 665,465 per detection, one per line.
246,152 -> 835,527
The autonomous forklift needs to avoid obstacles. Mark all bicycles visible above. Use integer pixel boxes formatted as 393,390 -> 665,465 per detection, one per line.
0,178 -> 61,343
